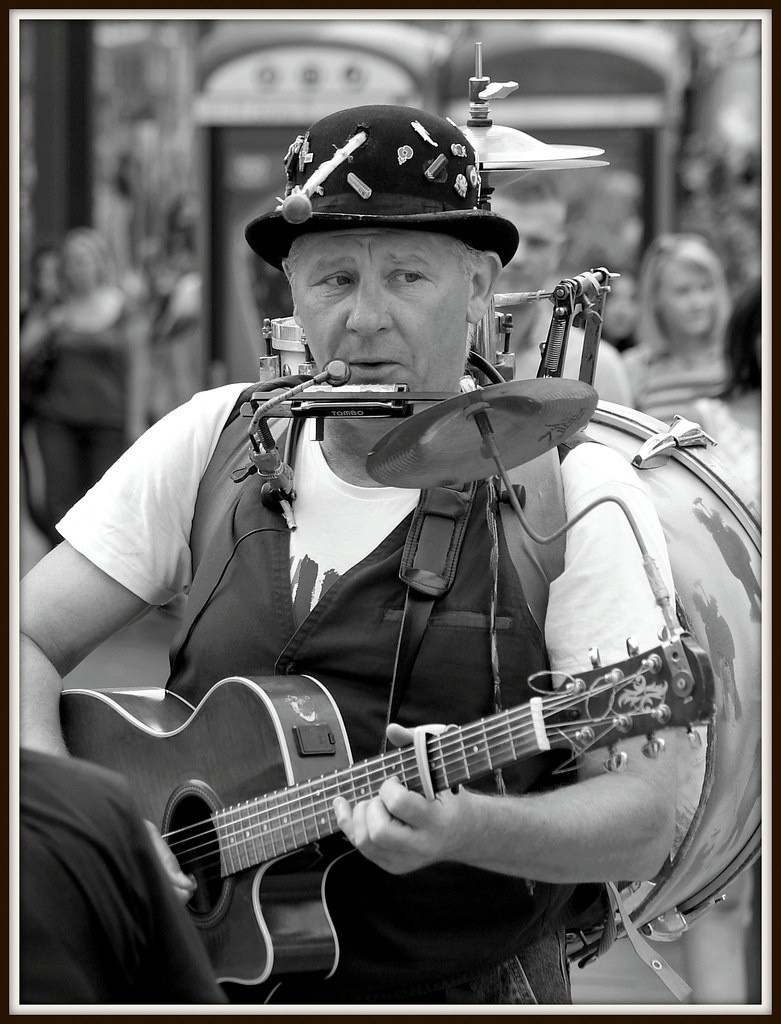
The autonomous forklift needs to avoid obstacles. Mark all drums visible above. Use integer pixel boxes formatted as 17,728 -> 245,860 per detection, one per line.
580,400 -> 760,942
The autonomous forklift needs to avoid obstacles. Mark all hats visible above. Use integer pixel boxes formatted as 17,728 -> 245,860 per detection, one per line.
245,105 -> 519,272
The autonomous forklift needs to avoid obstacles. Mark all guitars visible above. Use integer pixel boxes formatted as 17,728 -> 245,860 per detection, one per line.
52,636 -> 718,1005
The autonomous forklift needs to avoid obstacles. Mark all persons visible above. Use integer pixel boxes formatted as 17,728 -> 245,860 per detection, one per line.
18,748 -> 233,1007
21,159 -> 761,554
18,107 -> 687,1006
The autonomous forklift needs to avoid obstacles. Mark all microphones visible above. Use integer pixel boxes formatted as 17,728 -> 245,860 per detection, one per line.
249,358 -> 351,455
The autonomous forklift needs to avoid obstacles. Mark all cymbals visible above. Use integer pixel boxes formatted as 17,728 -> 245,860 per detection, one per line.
362,378 -> 600,491
456,122 -> 614,190
